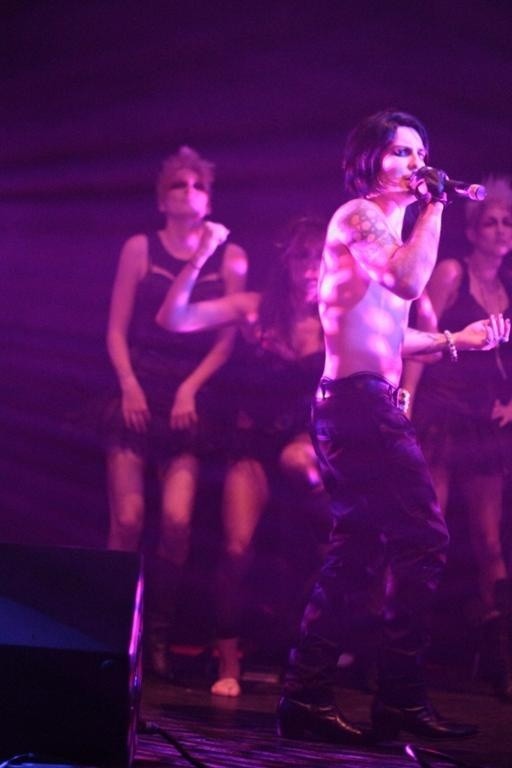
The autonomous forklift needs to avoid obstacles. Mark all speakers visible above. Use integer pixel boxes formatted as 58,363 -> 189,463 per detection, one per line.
0,541 -> 147,759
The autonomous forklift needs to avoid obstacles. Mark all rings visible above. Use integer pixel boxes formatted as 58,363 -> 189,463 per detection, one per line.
485,339 -> 492,345
500,337 -> 511,345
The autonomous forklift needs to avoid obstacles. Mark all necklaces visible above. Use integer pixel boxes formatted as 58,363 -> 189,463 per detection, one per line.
468,257 -> 506,318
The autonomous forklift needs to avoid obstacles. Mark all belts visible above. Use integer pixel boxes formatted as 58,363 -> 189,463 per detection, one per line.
314,377 -> 411,413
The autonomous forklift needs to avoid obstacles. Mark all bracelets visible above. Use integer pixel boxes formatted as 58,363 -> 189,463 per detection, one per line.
186,258 -> 203,272
445,327 -> 460,362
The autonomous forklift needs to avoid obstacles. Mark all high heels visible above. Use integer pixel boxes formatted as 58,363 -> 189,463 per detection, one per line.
275,694 -> 375,749
366,700 -> 482,747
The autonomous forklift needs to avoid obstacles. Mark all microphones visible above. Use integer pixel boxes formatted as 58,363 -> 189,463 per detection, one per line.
412,173 -> 486,202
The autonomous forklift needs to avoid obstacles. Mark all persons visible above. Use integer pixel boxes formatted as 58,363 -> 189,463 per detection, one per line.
98,144 -> 250,679
157,212 -> 442,694
276,111 -> 479,748
397,171 -> 512,710
